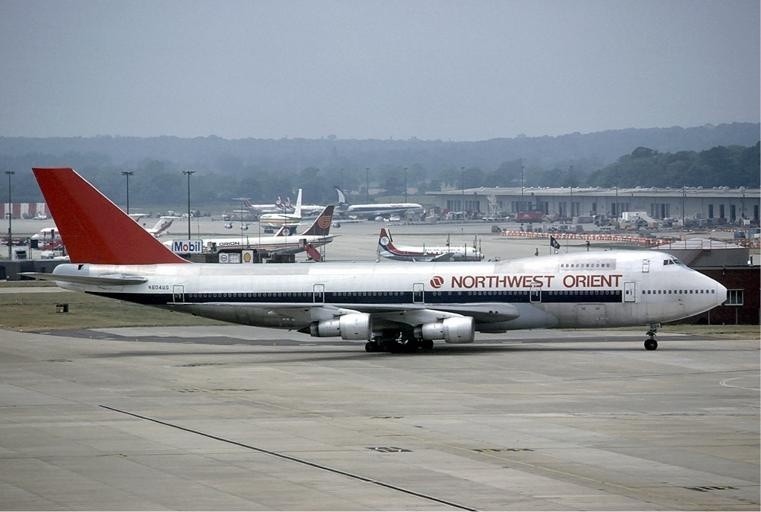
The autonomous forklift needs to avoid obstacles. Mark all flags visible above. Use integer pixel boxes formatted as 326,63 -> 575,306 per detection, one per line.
551,235 -> 560,249
